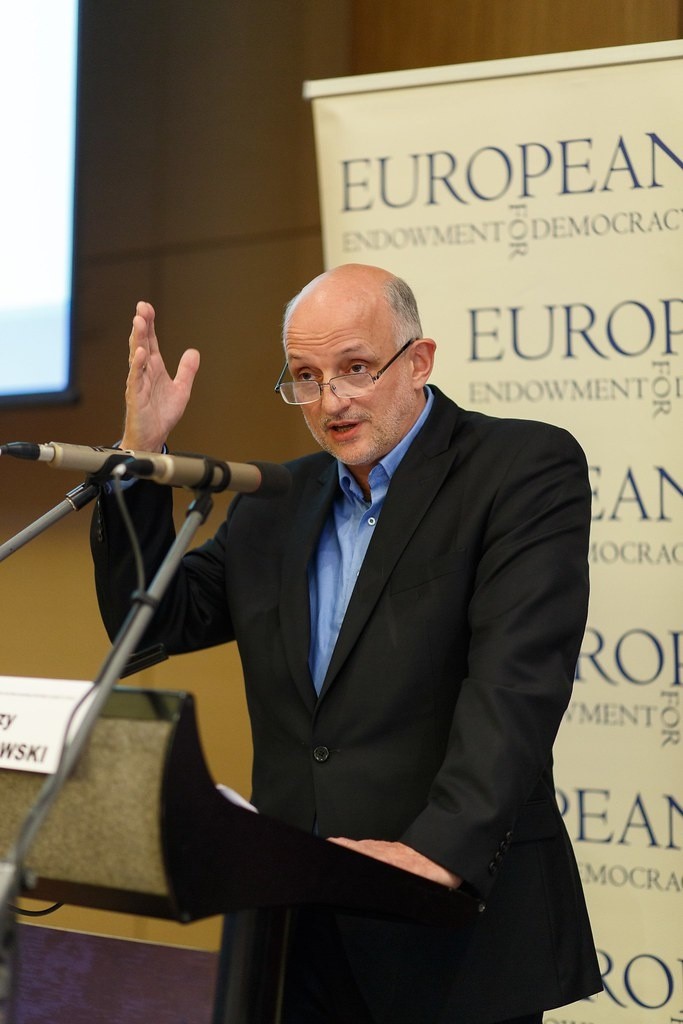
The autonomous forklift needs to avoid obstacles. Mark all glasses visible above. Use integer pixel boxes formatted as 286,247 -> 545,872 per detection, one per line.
274,337 -> 416,405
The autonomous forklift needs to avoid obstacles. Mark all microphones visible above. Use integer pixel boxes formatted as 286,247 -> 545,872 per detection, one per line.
4,440 -> 294,499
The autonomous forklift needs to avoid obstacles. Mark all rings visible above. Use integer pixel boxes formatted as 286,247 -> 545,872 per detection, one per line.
129,363 -> 146,370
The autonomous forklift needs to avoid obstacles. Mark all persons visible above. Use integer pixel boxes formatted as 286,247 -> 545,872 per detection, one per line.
90,263 -> 603,1024
